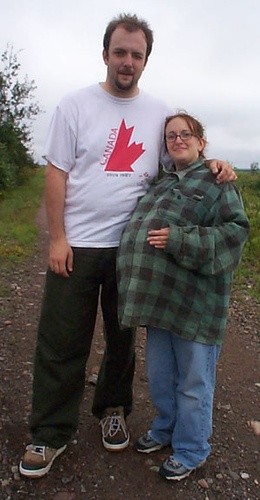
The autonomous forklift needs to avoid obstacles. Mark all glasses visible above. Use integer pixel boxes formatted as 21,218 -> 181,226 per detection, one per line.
167,132 -> 202,141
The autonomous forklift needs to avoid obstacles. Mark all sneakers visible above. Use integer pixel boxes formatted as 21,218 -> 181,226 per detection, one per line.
102,406 -> 131,452
160,455 -> 197,480
137,433 -> 165,453
19,444 -> 67,478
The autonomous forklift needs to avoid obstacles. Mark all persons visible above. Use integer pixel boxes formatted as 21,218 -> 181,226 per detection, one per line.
116,109 -> 252,482
19,11 -> 237,478
